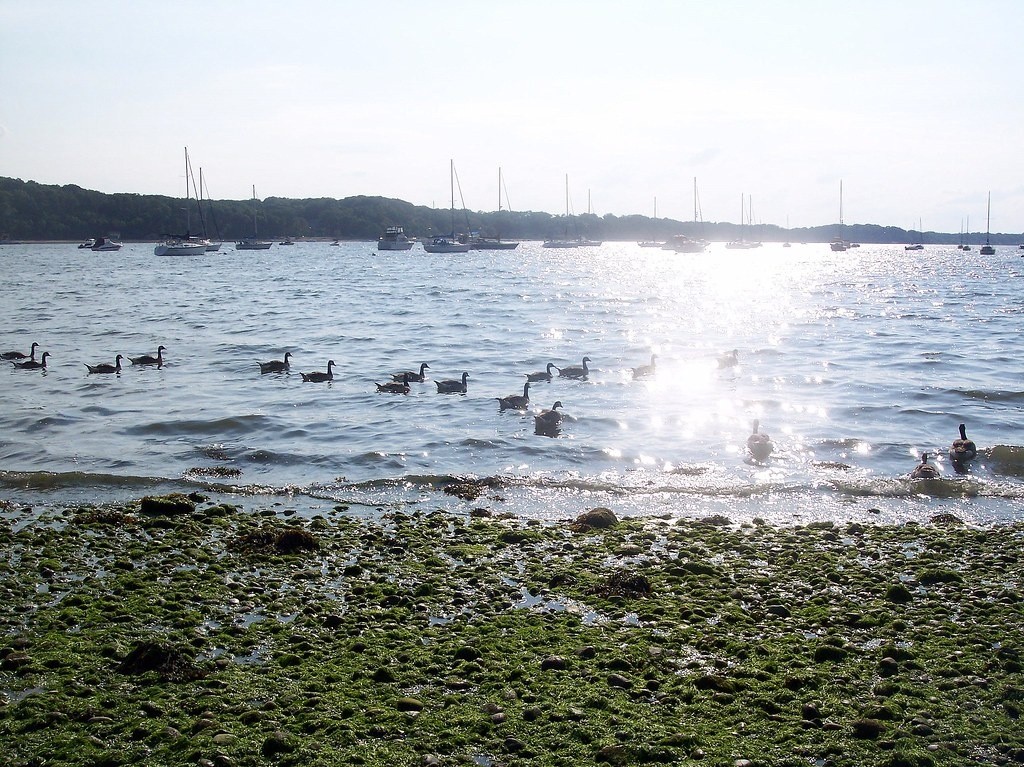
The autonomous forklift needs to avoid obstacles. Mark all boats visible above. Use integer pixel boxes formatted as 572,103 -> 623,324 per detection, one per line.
377,226 -> 415,251
279,240 -> 339,246
78,237 -> 124,251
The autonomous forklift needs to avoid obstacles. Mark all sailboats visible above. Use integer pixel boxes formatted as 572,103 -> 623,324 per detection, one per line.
154,146 -> 208,256
422,158 -> 475,252
662,177 -> 712,253
636,195 -> 663,247
979,190 -> 995,255
957,218 -> 963,249
458,166 -> 520,250
783,215 -> 791,247
915,217 -> 924,249
905,221 -> 918,250
542,173 -> 579,248
724,193 -> 763,249
572,188 -> 604,246
829,179 -> 861,251
963,215 -> 971,251
165,166 -> 222,251
236,184 -> 273,249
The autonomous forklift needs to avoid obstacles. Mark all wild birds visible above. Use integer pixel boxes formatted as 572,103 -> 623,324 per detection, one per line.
0,342 -> 52,370
714,348 -> 740,368
374,362 -> 471,395
629,353 -> 662,379
256,351 -> 336,383
83,345 -> 167,375
746,417 -> 775,461
910,423 -> 978,481
494,356 -> 591,431
